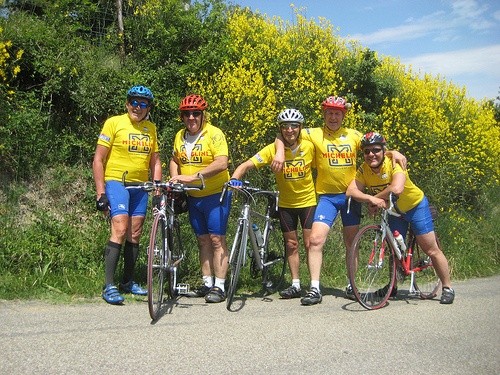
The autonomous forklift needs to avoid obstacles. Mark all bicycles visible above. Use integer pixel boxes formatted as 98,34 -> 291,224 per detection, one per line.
345,191 -> 441,311
217,179 -> 287,310
121,169 -> 205,321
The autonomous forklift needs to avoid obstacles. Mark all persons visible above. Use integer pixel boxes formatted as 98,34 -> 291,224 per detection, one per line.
346,132 -> 455,304
169,94 -> 233,302
93,85 -> 162,304
271,97 -> 407,304
228,110 -> 316,298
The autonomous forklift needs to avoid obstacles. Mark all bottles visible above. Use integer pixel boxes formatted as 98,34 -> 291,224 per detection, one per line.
98,201 -> 104,206
252,224 -> 263,246
393,230 -> 407,251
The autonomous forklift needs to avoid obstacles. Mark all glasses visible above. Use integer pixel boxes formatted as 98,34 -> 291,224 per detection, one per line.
129,100 -> 148,109
364,149 -> 382,154
183,112 -> 201,117
281,123 -> 300,128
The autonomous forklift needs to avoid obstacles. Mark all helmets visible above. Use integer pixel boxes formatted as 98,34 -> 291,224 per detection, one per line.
322,96 -> 347,113
359,132 -> 386,150
178,94 -> 208,110
277,109 -> 304,124
127,86 -> 154,103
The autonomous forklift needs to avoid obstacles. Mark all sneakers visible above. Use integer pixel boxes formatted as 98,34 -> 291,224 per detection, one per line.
301,287 -> 322,305
346,286 -> 360,300
279,286 -> 305,298
185,285 -> 211,298
205,286 -> 227,303
375,284 -> 397,297
102,284 -> 124,304
120,281 -> 148,295
440,287 -> 455,304
